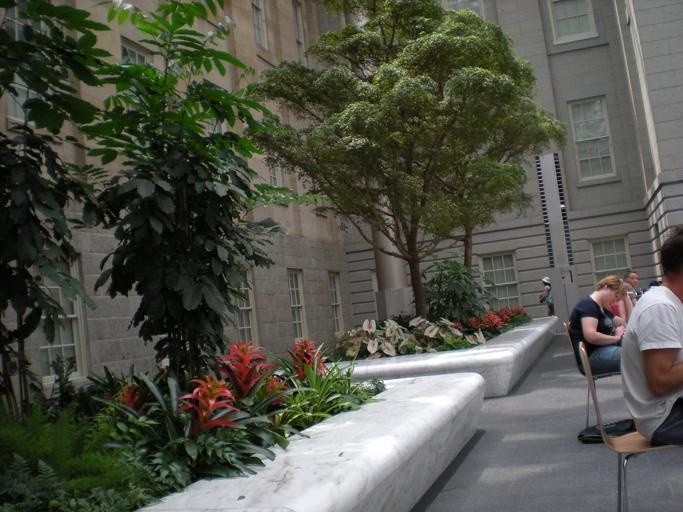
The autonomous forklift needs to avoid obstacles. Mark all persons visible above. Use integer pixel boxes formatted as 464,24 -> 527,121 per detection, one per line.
536,276 -> 554,316
567,227 -> 683,447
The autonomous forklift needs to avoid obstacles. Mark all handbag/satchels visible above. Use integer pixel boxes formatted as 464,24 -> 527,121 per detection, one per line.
538,295 -> 546,303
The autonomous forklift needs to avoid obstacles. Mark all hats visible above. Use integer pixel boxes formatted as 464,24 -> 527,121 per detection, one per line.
542,277 -> 551,285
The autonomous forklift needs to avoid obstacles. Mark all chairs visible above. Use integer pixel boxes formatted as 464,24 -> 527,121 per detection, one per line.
564,321 -> 682,512
